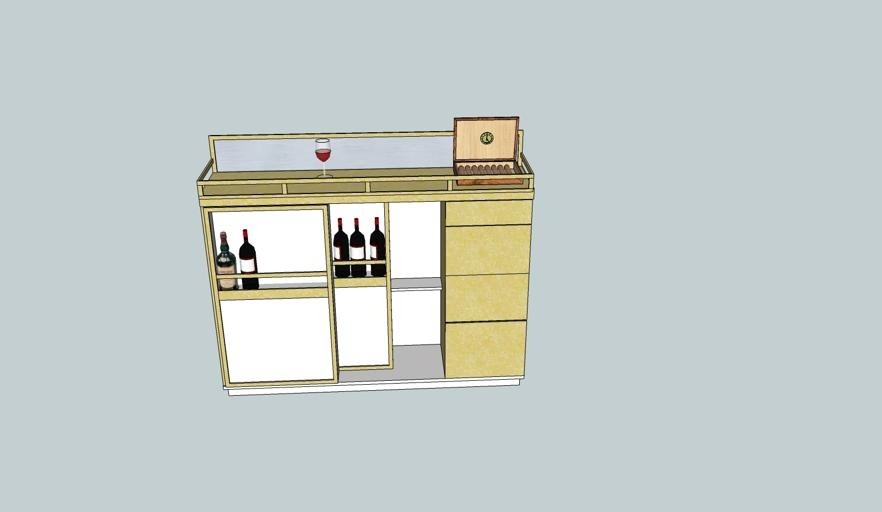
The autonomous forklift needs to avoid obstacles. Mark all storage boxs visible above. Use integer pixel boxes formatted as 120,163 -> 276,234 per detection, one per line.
453,117 -> 524,185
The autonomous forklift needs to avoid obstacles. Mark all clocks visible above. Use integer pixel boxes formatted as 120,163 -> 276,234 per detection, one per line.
481,132 -> 494,145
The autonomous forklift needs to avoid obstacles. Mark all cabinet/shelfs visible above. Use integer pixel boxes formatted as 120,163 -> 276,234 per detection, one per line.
194,130 -> 535,397
